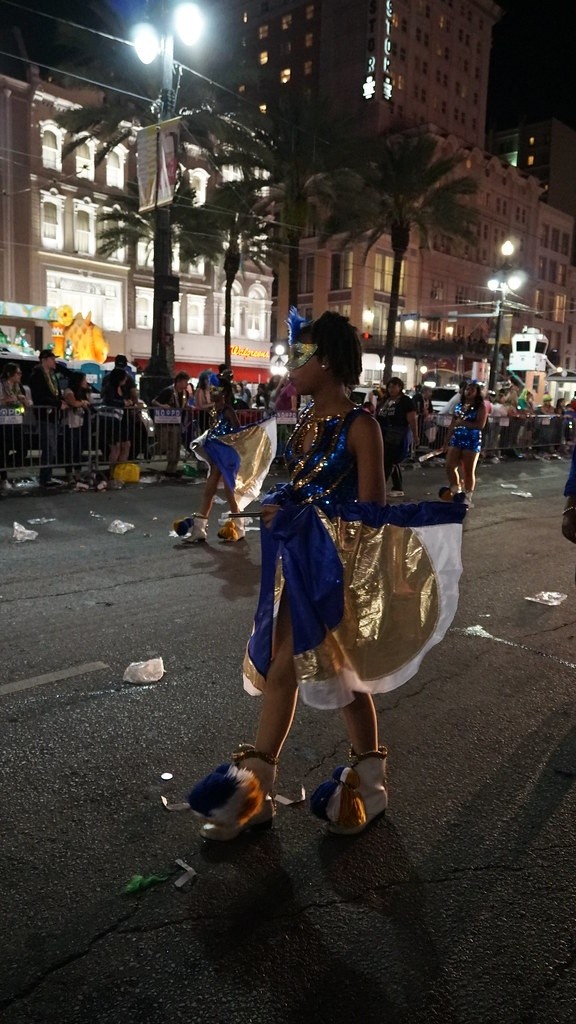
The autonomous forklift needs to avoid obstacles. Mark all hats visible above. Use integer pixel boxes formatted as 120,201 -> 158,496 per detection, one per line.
38,349 -> 60,359
542,393 -> 552,402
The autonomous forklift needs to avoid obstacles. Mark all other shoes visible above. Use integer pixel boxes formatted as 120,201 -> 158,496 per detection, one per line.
144,446 -> 152,464
67,479 -> 77,487
39,480 -> 63,490
165,473 -> 178,479
76,477 -> 88,484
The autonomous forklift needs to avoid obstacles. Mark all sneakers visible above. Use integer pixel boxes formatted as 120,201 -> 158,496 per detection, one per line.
387,490 -> 405,498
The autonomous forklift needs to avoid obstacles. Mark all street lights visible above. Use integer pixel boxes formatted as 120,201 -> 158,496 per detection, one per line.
274,345 -> 285,379
133,2 -> 210,402
487,239 -> 527,396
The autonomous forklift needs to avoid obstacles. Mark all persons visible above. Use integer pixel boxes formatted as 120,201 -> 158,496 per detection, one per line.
440,380 -> 576,510
151,363 -> 305,543
189,308 -> 468,841
0,349 -> 154,491
368,374 -> 438,496
562,445 -> 576,584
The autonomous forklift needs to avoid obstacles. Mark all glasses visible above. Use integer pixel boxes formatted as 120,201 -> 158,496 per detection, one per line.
16,371 -> 23,376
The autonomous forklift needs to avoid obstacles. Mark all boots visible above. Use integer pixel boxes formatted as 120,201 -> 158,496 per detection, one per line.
186,742 -> 280,842
173,512 -> 209,544
437,484 -> 473,507
217,517 -> 245,542
309,744 -> 388,836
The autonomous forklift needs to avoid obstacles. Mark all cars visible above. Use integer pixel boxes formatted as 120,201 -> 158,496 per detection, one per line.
351,388 -> 380,412
1,349 -> 155,461
428,386 -> 458,413
